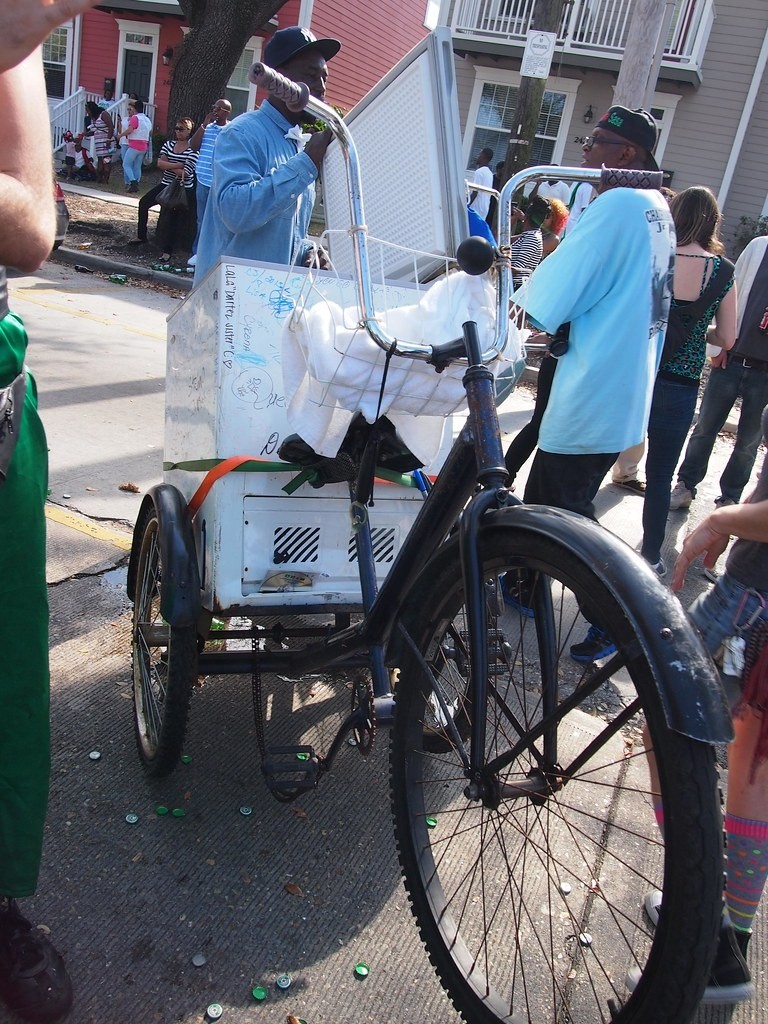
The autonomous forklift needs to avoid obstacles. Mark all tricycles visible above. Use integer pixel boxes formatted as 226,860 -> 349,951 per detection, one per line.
123,62 -> 740,1024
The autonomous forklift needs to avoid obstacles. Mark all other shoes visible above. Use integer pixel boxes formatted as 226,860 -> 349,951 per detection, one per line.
655,559 -> 667,577
158,252 -> 171,263
127,178 -> 140,193
706,567 -> 724,584
128,237 -> 149,244
613,479 -> 647,498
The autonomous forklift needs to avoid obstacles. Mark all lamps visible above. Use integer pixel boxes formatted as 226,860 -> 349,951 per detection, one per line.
161,48 -> 173,65
584,105 -> 593,123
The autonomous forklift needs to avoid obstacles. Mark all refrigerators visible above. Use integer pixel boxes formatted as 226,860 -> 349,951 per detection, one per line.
160,24 -> 475,614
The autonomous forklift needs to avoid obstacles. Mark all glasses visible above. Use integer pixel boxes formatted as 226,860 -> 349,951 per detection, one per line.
583,135 -> 629,148
175,126 -> 186,131
127,107 -> 133,110
212,104 -> 231,112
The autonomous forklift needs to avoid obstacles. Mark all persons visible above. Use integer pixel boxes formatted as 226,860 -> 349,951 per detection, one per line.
625,448 -> 768,1005
65,131 -> 75,181
505,187 -> 677,491
117,102 -> 136,189
640,186 -> 738,577
0,0 -> 98,1024
498,104 -> 678,662
187,99 -> 232,265
128,117 -> 200,262
540,198 -> 569,265
82,101 -> 114,184
511,195 -> 551,292
98,89 -> 115,108
670,235 -> 768,509
130,93 -> 137,99
191,25 -> 341,289
116,102 -> 152,193
466,147 -> 493,221
529,164 -> 570,207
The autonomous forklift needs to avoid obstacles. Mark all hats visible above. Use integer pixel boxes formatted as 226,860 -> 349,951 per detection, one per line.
263,26 -> 342,70
595,105 -> 659,172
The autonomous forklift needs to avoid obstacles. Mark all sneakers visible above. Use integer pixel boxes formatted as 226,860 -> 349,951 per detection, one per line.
499,573 -> 537,618
625,924 -> 754,1004
0,896 -> 73,1024
715,496 -> 737,512
668,481 -> 693,510
187,254 -> 197,265
569,625 -> 618,662
644,888 -> 753,965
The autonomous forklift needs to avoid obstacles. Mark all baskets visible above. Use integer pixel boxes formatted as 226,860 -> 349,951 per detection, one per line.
279,229 -> 532,418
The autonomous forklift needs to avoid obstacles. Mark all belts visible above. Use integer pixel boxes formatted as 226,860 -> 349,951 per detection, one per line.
727,353 -> 768,369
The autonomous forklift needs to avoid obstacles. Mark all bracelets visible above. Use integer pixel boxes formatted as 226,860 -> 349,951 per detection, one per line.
201,124 -> 206,130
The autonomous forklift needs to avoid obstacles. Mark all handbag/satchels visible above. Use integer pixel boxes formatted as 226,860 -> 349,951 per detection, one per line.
155,169 -> 190,214
